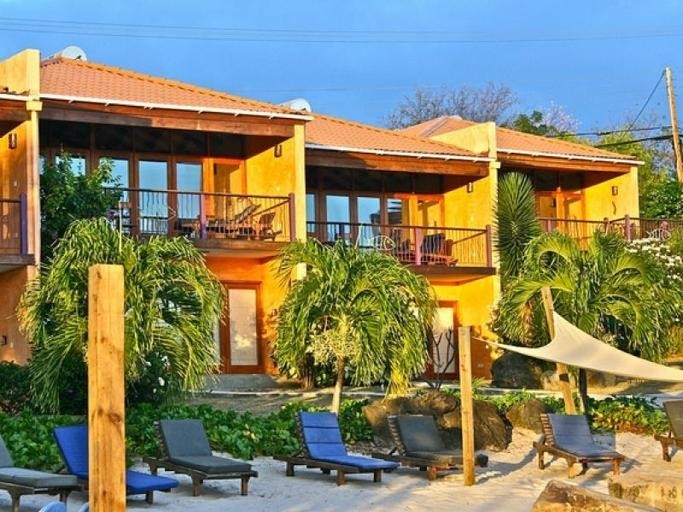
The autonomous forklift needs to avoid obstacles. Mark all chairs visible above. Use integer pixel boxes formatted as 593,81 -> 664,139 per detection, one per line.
173,199 -> 276,242
333,226 -> 459,268
